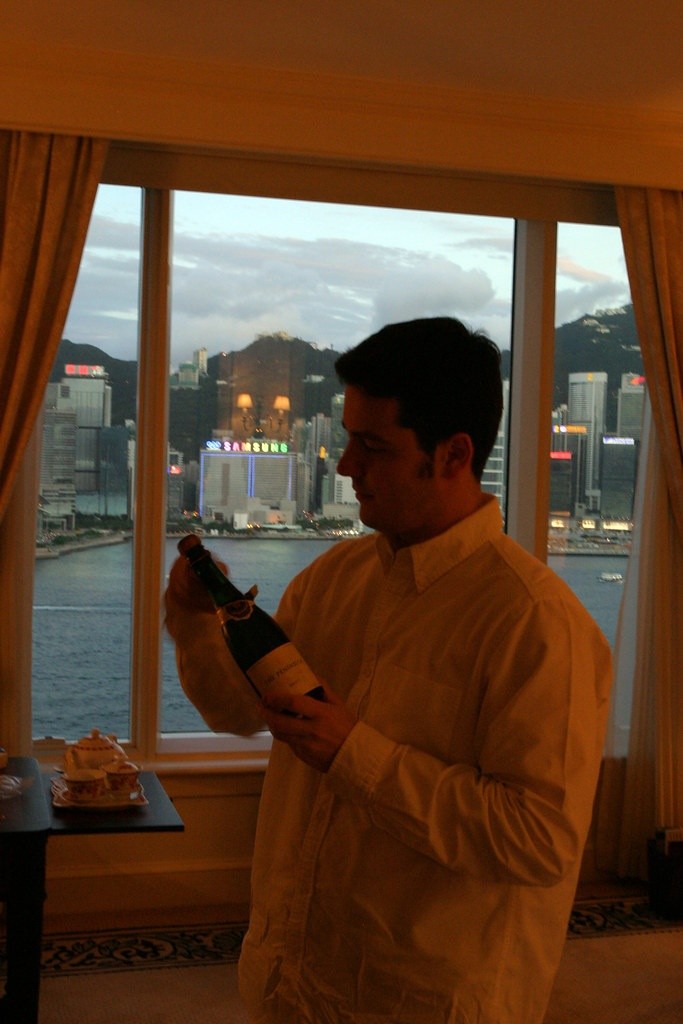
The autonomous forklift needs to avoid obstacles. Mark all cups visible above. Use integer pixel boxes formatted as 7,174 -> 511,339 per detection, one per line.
60,769 -> 106,801
102,760 -> 143,794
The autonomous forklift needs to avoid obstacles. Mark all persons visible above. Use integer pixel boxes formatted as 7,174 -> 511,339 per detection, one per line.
165,318 -> 613,1023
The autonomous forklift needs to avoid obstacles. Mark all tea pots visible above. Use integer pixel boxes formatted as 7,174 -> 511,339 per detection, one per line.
66,729 -> 128,768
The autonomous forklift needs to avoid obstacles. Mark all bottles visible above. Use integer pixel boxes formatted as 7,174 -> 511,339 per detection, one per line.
655,832 -> 667,855
177,535 -> 328,717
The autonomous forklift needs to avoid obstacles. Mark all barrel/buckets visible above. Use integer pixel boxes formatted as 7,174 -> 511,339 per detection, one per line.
647,841 -> 683,919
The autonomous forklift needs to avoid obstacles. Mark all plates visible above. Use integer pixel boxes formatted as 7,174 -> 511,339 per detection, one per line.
60,784 -> 145,804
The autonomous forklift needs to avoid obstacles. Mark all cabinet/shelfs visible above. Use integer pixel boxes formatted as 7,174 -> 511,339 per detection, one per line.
0,756 -> 185,1024
